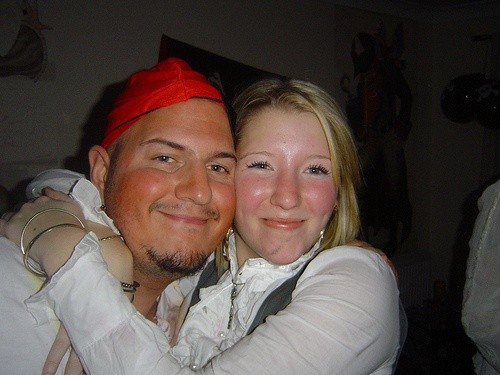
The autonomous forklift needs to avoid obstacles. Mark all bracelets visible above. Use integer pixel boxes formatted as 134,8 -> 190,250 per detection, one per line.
23,224 -> 88,277
119,281 -> 140,303
20,208 -> 87,274
97,235 -> 123,242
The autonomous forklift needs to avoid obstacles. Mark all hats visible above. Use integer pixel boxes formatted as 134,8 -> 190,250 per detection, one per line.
101,57 -> 227,147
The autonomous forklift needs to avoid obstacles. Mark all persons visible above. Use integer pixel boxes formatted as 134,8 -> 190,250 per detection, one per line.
0,57 -> 236,375
0,78 -> 408,375
